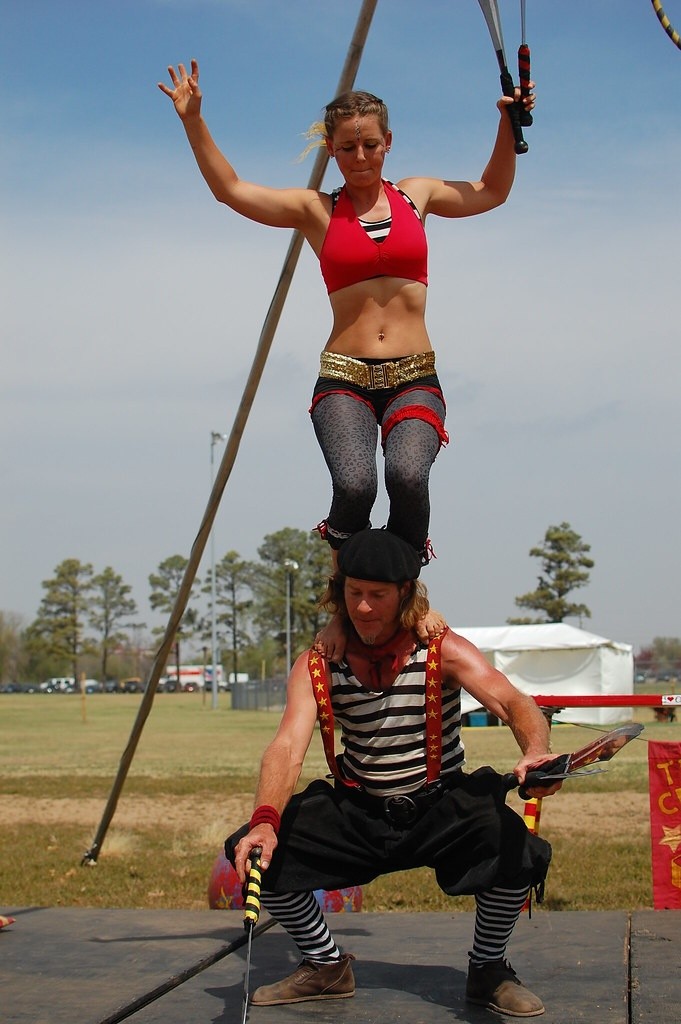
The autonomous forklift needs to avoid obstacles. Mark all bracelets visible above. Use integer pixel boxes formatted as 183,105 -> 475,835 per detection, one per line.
249,805 -> 281,835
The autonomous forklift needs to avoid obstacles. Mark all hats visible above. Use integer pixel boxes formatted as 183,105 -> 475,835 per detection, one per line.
337,524 -> 422,582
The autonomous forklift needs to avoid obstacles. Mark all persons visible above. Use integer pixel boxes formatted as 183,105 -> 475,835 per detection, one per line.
158,59 -> 537,663
223,528 -> 563,1016
654,707 -> 675,722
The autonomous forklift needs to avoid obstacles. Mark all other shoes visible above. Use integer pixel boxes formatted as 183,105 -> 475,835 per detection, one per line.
465,959 -> 542,1015
250,953 -> 355,1004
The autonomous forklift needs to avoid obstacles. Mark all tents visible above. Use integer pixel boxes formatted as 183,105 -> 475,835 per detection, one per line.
450,623 -> 634,726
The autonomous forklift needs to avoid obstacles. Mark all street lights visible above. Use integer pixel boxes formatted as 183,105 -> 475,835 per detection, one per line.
281,555 -> 299,678
208,428 -> 228,711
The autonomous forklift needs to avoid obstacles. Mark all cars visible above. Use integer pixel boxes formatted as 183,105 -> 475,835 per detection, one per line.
0,665 -> 249,693
